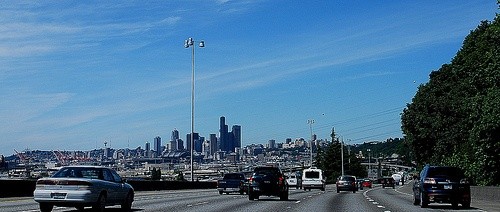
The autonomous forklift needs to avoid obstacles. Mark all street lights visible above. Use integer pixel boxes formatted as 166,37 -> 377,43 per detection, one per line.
306,119 -> 314,167
345,138 -> 352,175
367,149 -> 371,173
184,38 -> 205,181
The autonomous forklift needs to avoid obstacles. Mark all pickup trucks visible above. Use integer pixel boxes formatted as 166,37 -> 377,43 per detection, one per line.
217,172 -> 246,196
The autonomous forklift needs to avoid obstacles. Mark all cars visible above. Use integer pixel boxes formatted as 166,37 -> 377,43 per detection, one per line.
412,165 -> 472,211
34,165 -> 135,212
392,172 -> 414,186
336,176 -> 357,195
381,176 -> 397,190
355,179 -> 372,190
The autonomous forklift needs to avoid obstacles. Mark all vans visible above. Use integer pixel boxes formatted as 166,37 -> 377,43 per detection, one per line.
285,172 -> 300,189
248,166 -> 289,201
302,167 -> 326,193
